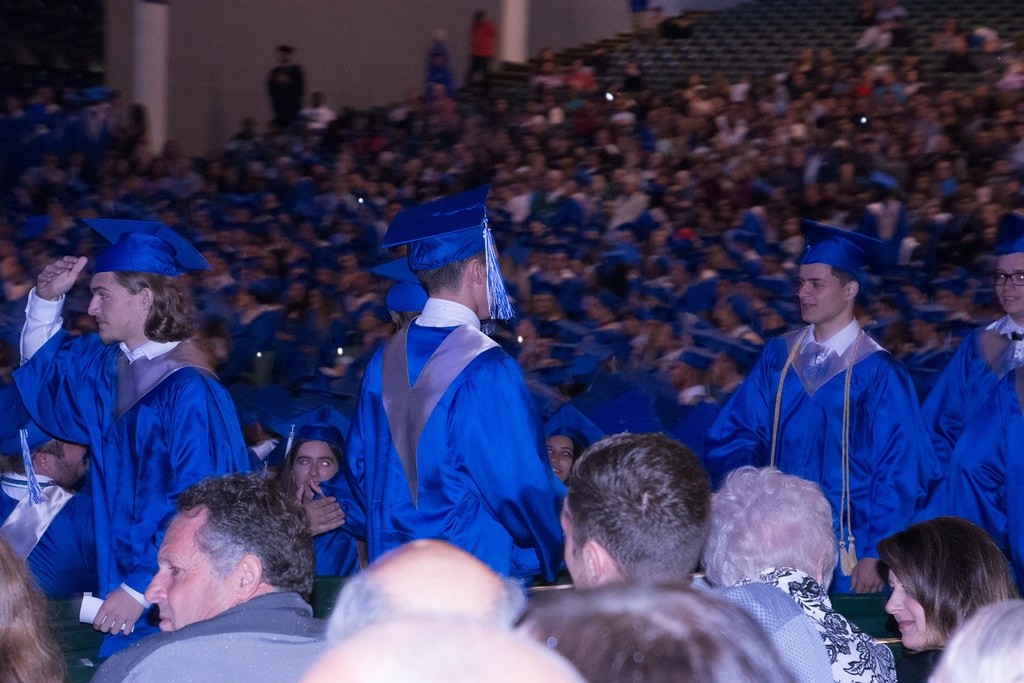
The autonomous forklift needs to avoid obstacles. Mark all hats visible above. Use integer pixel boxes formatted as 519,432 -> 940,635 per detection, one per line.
190,190 -> 391,337
82,217 -> 211,278
987,212 -> 1024,256
861,172 -> 1000,342
271,404 -> 352,462
0,217 -> 93,355
493,180 -> 800,384
380,184 -> 513,321
0,382 -> 54,507
543,403 -> 607,449
369,257 -> 428,313
796,221 -> 895,299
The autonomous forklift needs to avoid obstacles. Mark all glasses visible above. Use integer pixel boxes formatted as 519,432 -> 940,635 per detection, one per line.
989,272 -> 1024,287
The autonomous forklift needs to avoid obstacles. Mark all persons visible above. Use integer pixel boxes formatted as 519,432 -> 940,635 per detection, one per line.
247,419 -> 283,461
299,538 -> 593,683
537,401 -> 609,483
346,179 -> 574,588
704,219 -> 946,593
11,219 -> 253,660
516,431 -> 900,683
284,387 -> 357,505
0,436 -> 100,603
90,470 -> 328,683
1,0 -> 1024,409
919,214 -> 1024,599
0,538 -> 67,682
876,516 -> 1024,683
